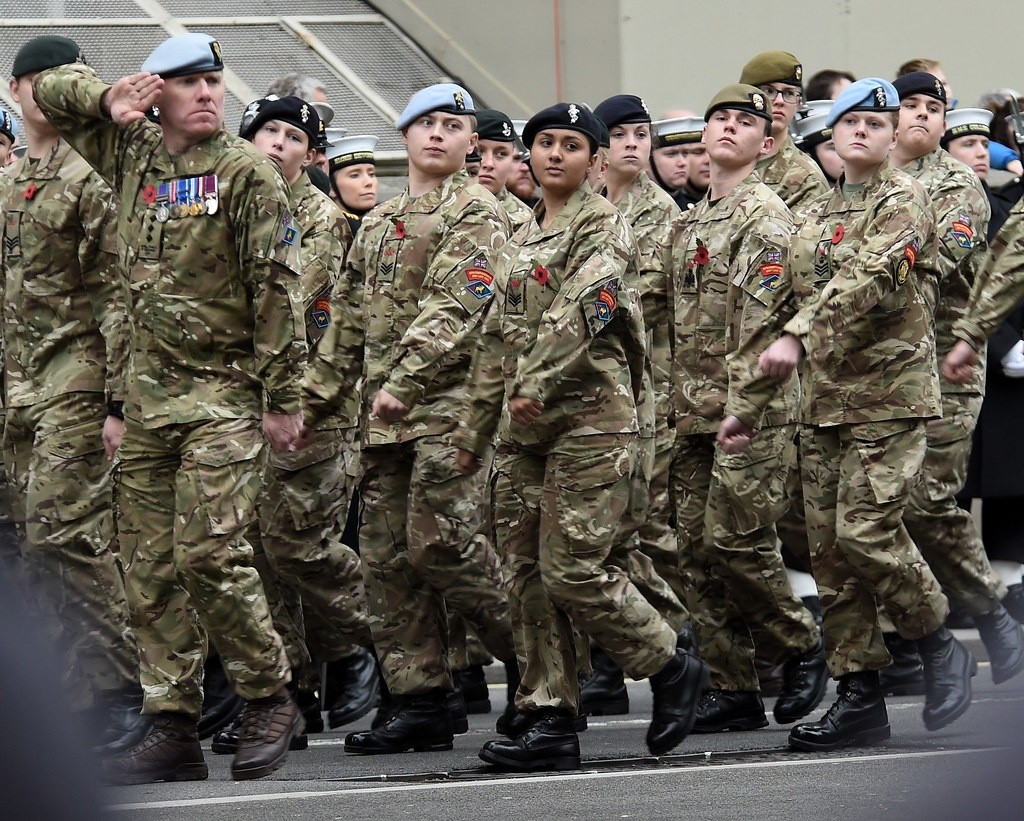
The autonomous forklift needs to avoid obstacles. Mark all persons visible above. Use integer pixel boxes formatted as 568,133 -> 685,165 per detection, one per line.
643,84 -> 831,731
450,103 -> 709,767
717,77 -> 977,754
0,33 -> 1024,785
297,83 -> 593,754
30,34 -> 309,779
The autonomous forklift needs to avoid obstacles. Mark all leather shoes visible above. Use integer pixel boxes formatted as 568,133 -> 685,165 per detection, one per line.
559,671 -> 629,715
917,622 -> 979,732
689,687 -> 770,735
645,652 -> 706,755
773,641 -> 829,723
833,640 -> 925,696
83,652 -> 492,786
975,603 -> 1024,685
478,705 -> 581,771
786,671 -> 891,752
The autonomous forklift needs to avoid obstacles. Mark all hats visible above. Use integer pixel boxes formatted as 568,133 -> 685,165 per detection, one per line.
889,72 -> 947,102
940,109 -> 994,146
239,52 -> 836,182
140,33 -> 224,78
825,78 -> 900,127
0,107 -> 18,144
11,35 -> 87,77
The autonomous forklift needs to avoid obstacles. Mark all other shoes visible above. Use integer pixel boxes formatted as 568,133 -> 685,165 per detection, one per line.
943,579 -> 1024,628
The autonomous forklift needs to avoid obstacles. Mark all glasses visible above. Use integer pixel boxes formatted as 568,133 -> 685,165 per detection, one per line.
758,85 -> 806,105
